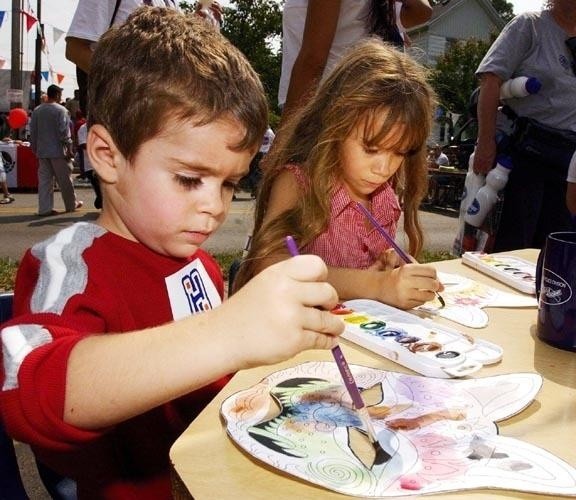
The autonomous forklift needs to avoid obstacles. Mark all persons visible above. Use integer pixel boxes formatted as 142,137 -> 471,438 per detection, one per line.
421,144 -> 452,205
0,6 -> 348,500
566,150 -> 576,217
474,0 -> 576,254
230,38 -> 445,310
258,0 -> 433,171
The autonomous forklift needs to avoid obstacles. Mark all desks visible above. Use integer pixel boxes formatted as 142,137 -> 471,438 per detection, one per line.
168,244 -> 574,499
424,163 -> 470,215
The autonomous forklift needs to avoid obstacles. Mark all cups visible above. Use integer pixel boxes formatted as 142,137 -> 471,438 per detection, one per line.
535,229 -> 576,351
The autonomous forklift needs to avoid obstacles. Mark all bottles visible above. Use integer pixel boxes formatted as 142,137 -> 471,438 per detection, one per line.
499,76 -> 540,102
461,143 -> 484,213
464,153 -> 514,229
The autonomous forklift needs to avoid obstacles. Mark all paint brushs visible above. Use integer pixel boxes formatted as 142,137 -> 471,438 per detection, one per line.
286,235 -> 392,465
356,201 -> 446,307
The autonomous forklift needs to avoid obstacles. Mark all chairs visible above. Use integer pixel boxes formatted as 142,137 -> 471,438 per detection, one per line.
0,286 -> 75,500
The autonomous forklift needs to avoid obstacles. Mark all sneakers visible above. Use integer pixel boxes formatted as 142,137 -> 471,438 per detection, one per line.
77,201 -> 83,208
50,210 -> 57,215
0,194 -> 15,203
